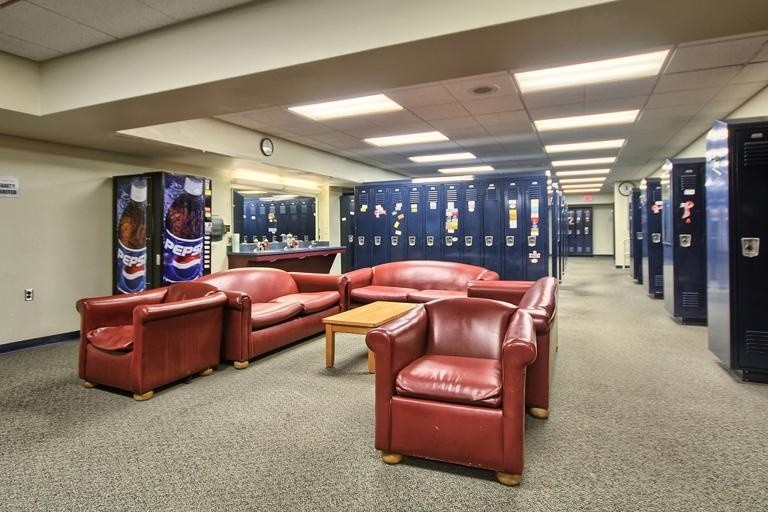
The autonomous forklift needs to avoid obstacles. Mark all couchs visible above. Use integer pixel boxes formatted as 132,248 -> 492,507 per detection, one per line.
197,261 -> 499,369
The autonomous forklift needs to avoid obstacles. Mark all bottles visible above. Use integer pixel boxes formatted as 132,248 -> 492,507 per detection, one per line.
114,179 -> 148,294
163,177 -> 205,286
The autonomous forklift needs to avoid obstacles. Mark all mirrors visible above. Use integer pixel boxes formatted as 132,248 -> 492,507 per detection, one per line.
230,188 -> 317,243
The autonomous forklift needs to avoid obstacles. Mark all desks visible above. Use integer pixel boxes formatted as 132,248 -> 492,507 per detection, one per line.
227,245 -> 346,273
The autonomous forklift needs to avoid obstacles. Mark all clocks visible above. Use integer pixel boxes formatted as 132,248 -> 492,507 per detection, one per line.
618,180 -> 636,196
261,138 -> 275,156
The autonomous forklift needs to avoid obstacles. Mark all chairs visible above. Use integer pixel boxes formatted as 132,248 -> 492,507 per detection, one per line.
73,281 -> 228,401
367,278 -> 555,487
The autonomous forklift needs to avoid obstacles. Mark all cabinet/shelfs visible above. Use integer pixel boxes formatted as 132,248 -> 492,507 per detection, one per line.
338,169 -> 594,284
628,115 -> 768,384
232,192 -> 315,243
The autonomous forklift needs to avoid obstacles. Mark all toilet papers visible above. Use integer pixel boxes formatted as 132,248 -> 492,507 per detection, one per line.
231,233 -> 240,253
